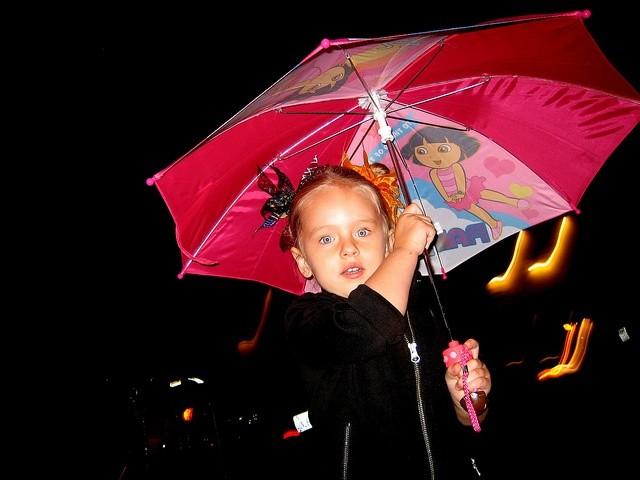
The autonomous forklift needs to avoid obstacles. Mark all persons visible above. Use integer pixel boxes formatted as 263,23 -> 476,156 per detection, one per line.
218,57 -> 355,132
400,126 -> 531,240
250,163 -> 493,479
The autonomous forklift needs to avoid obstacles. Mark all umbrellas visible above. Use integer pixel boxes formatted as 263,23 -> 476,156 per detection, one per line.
145,8 -> 640,421
